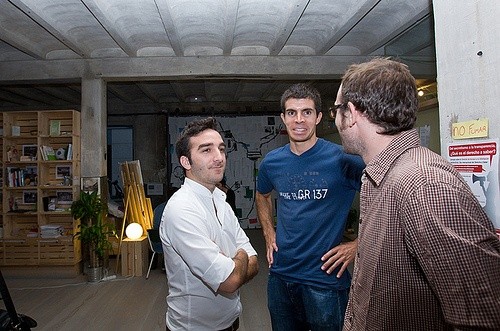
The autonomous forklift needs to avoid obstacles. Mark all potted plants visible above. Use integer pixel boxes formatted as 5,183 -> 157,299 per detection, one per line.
345,208 -> 357,234
64,187 -> 119,282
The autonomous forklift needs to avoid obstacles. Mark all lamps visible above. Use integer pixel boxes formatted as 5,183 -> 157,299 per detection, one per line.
126,223 -> 144,240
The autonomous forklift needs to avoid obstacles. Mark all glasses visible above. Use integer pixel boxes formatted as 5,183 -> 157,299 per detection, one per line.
328,103 -> 347,118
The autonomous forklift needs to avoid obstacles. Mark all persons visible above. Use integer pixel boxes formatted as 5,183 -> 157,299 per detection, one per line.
328,55 -> 500,331
154,118 -> 260,331
255,84 -> 366,331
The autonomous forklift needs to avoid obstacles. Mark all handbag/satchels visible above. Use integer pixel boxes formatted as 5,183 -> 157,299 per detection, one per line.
0,309 -> 37,331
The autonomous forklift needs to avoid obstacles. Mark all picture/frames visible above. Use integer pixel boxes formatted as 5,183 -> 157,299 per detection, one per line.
22,144 -> 37,156
55,190 -> 73,205
55,164 -> 71,179
22,190 -> 37,205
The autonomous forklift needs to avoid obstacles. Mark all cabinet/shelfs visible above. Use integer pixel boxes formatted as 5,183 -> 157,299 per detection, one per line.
0,109 -> 82,274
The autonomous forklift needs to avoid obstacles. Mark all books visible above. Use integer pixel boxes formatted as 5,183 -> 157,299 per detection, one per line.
5,167 -> 25,187
39,144 -> 72,161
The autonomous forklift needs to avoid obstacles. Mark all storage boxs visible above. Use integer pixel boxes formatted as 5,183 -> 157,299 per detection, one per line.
144,181 -> 163,196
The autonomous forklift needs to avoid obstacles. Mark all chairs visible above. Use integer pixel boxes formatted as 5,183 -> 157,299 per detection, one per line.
145,228 -> 166,279
